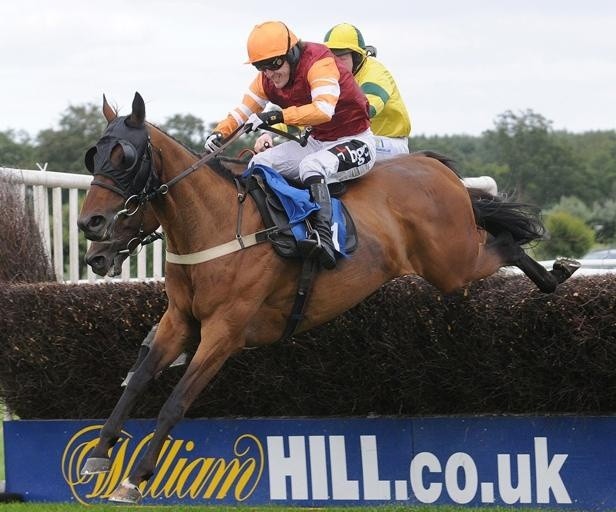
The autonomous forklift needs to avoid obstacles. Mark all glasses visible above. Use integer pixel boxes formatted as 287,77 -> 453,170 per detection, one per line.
252,58 -> 286,70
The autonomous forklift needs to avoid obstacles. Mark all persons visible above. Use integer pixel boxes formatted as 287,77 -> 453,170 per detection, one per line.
250,21 -> 415,171
201,19 -> 377,276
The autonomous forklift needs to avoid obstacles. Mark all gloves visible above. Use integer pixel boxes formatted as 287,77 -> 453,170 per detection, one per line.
243,110 -> 284,134
203,132 -> 226,155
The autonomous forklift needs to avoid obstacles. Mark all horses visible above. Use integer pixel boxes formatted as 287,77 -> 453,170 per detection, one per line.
78,90 -> 582,503
81,152 -> 251,387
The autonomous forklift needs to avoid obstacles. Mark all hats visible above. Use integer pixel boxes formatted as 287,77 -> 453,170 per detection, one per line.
243,18 -> 300,65
322,22 -> 367,56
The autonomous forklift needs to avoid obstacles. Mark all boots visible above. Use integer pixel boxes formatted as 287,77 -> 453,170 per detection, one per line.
297,183 -> 337,269
